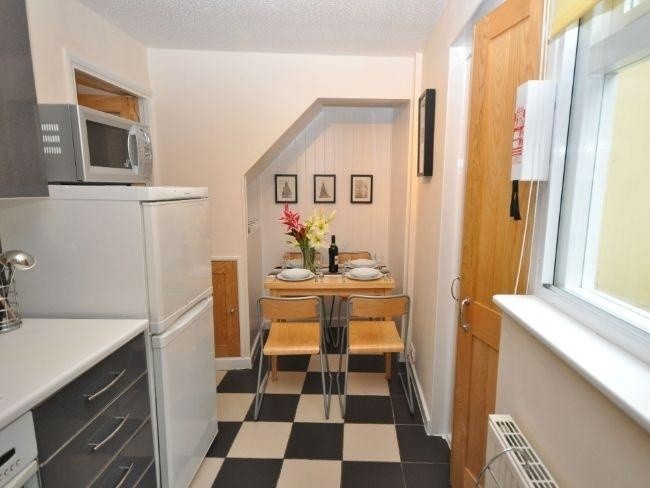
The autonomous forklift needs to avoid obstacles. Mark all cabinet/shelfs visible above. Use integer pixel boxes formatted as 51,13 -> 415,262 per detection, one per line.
1,0 -> 52,201
28,330 -> 160,487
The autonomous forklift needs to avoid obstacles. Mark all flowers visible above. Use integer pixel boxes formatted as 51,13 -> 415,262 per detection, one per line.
279,204 -> 337,271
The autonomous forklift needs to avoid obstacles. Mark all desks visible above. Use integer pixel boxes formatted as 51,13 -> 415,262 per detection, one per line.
261,264 -> 396,385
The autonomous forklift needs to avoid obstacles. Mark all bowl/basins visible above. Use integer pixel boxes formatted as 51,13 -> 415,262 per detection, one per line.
351,259 -> 376,277
282,269 -> 312,278
286,258 -> 303,267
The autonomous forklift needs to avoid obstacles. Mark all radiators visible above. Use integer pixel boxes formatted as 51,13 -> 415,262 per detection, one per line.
477,412 -> 557,488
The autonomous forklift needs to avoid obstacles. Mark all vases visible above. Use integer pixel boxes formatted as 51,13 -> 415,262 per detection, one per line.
300,246 -> 316,273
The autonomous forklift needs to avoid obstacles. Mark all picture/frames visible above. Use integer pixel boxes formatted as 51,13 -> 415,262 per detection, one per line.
416,88 -> 435,177
350,173 -> 373,204
274,173 -> 298,204
313,174 -> 336,203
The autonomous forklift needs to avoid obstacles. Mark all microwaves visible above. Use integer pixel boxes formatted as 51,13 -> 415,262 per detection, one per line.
37,103 -> 154,185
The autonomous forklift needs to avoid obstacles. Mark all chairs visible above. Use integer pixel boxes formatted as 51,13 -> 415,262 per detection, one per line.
334,251 -> 372,346
253,293 -> 333,422
337,292 -> 417,417
277,249 -> 331,344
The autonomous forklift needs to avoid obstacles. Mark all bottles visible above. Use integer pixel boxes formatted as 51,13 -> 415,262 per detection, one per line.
328,236 -> 339,273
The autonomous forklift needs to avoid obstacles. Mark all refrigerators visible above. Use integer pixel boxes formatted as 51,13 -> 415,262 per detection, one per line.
1,184 -> 221,488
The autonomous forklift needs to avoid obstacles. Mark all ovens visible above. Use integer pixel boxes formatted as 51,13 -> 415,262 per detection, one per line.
2,402 -> 46,488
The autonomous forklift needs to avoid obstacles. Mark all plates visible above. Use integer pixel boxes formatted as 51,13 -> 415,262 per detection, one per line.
276,271 -> 316,281
344,258 -> 380,268
346,268 -> 383,280
